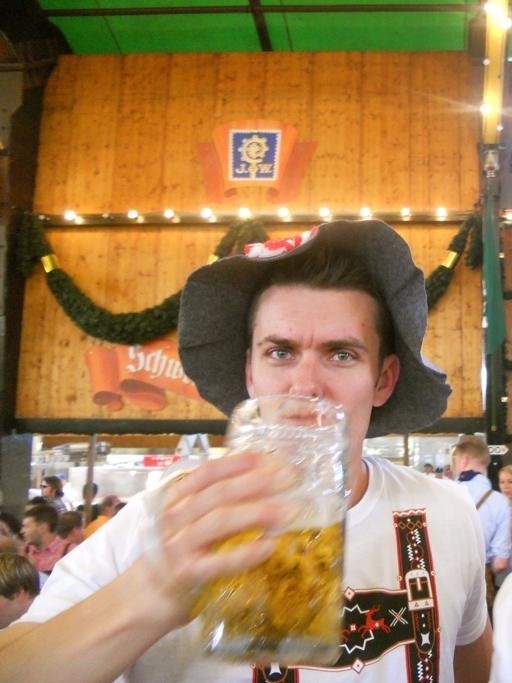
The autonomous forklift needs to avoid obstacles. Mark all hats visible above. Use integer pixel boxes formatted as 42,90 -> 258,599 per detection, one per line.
178,218 -> 452,438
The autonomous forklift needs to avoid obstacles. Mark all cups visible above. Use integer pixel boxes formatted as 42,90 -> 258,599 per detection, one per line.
207,394 -> 349,664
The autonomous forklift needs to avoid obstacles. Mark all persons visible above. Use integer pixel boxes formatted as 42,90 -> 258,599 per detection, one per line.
424,435 -> 512,590
0,219 -> 493,683
0,476 -> 127,635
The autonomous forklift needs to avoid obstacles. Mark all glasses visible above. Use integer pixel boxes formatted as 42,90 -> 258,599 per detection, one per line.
40,485 -> 47,489
